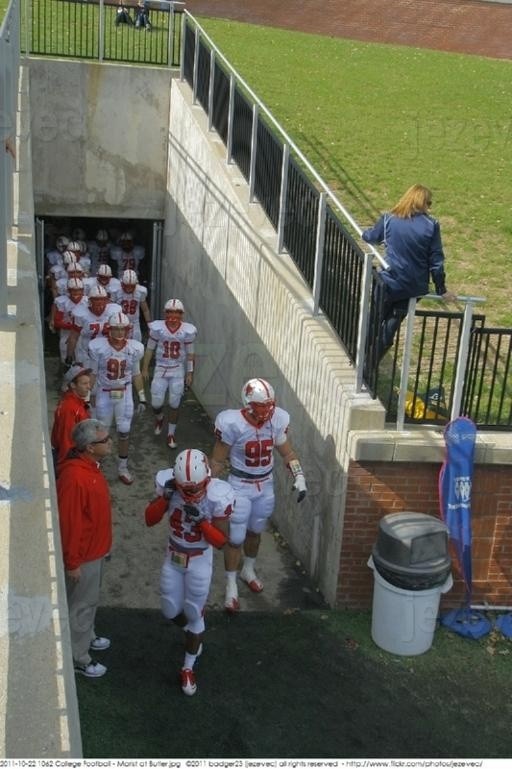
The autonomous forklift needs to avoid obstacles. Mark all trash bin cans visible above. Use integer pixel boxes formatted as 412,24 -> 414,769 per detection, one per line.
367,511 -> 454,657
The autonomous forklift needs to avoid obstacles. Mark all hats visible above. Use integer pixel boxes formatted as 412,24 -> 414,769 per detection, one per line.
63,364 -> 93,388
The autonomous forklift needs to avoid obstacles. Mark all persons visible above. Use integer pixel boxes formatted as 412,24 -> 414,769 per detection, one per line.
115,1 -> 134,27
208,377 -> 306,616
146,449 -> 237,698
56,419 -> 113,678
45,220 -> 195,483
351,184 -> 456,386
135,0 -> 153,30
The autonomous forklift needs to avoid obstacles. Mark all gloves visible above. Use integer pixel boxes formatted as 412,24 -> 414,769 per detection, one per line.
290,474 -> 309,504
182,499 -> 206,522
63,355 -> 76,369
137,401 -> 147,417
163,477 -> 176,501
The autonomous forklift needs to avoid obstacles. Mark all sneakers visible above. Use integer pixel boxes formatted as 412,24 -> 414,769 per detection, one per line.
180,623 -> 205,697
71,632 -> 111,679
154,420 -> 165,435
166,434 -> 179,450
238,566 -> 265,594
223,582 -> 240,614
117,465 -> 134,486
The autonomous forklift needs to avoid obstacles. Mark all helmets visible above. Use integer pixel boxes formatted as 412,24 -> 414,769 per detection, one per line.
87,286 -> 109,300
120,268 -> 139,286
96,263 -> 113,278
174,448 -> 212,492
106,313 -> 130,331
67,278 -> 85,291
45,217 -> 136,272
240,377 -> 276,415
162,298 -> 185,315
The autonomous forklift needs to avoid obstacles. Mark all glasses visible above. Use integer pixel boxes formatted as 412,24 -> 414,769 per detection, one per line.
86,431 -> 114,445
